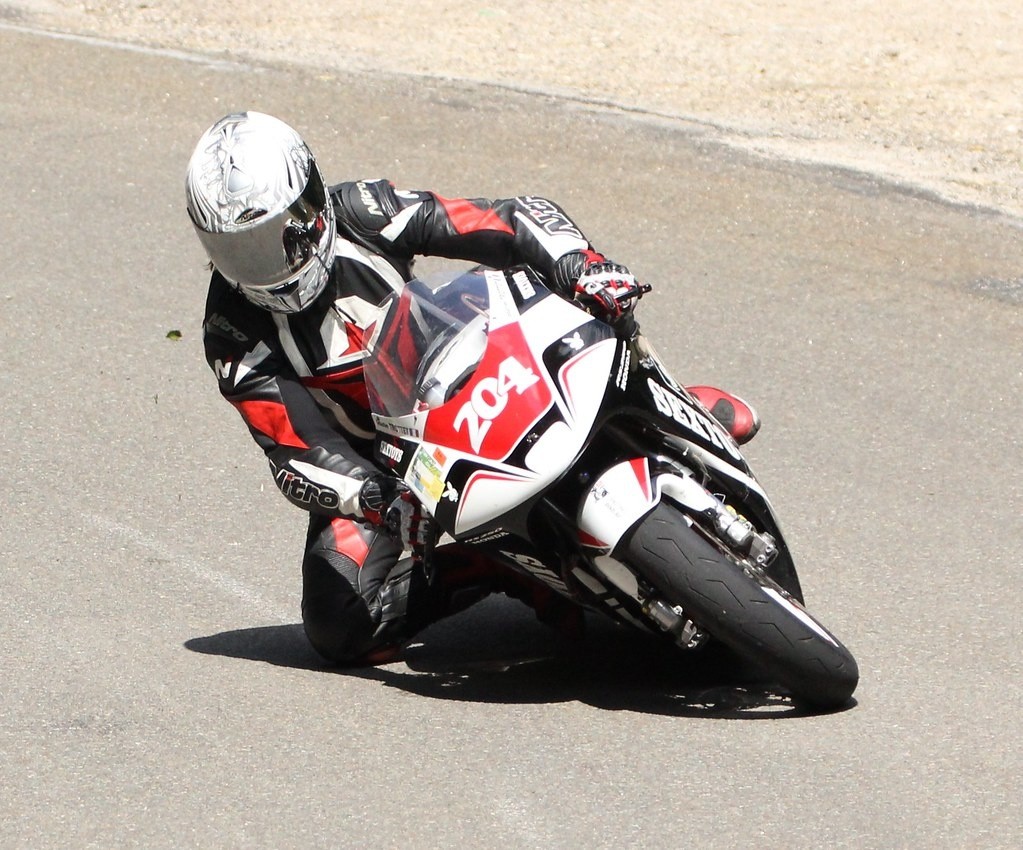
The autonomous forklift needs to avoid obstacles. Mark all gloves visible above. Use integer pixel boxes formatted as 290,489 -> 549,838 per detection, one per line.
359,474 -> 434,560
566,260 -> 641,326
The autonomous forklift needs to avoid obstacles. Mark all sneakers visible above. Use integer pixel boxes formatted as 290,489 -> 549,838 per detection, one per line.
518,579 -> 585,641
683,385 -> 761,445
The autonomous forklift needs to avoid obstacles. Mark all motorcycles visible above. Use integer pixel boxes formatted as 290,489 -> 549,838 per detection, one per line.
356,260 -> 860,712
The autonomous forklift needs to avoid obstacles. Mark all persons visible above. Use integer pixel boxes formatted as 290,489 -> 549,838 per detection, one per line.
183,109 -> 760,675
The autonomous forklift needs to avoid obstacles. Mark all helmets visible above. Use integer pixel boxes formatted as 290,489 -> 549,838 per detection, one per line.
183,111 -> 339,315
